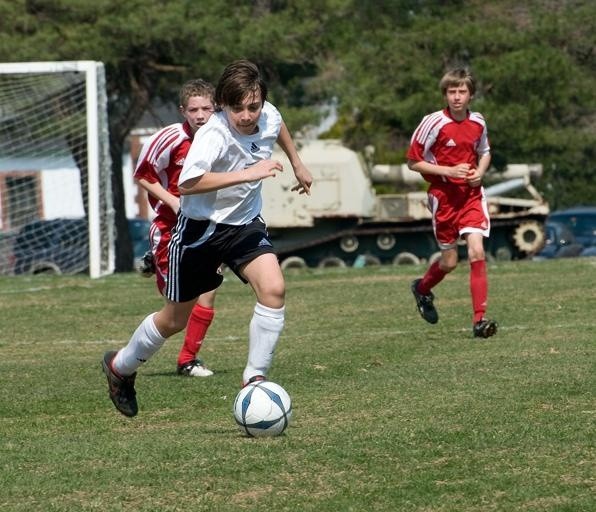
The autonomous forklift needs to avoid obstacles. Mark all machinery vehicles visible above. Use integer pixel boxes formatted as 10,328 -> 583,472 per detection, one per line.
127,126 -> 551,277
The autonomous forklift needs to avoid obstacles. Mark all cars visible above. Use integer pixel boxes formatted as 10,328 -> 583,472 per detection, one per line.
4,215 -> 155,281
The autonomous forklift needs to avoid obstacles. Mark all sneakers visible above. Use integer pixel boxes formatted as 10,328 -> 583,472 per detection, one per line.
100,351 -> 138,418
176,359 -> 214,376
137,250 -> 156,279
473,320 -> 497,339
411,278 -> 439,325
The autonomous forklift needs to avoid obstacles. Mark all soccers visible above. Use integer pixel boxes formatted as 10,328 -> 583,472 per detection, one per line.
232,380 -> 291,438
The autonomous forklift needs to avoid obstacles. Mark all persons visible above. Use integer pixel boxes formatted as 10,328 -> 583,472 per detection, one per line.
101,58 -> 312,419
134,77 -> 222,378
405,67 -> 499,339
